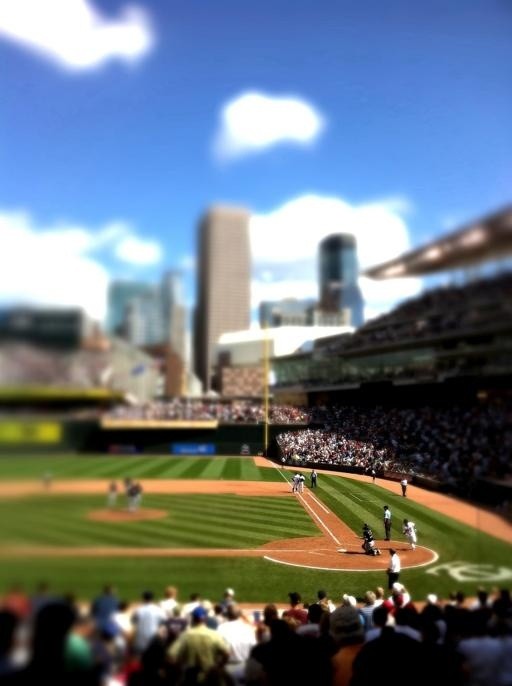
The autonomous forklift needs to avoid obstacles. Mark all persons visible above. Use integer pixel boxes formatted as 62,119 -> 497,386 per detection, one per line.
124,473 -> 132,497
291,471 -> 299,493
127,484 -> 138,512
309,469 -> 318,489
280,390 -> 511,482
313,273 -> 512,352
1,583 -> 512,685
360,526 -> 381,556
387,547 -> 401,587
299,473 -> 306,494
135,481 -> 143,505
112,399 -> 312,424
383,505 -> 394,542
403,518 -> 416,551
108,480 -> 117,506
401,473 -> 407,496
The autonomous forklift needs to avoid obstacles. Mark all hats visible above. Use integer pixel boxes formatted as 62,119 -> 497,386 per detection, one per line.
342,594 -> 357,608
328,606 -> 363,640
192,607 -> 207,619
